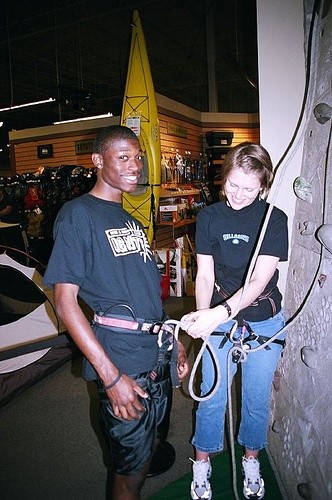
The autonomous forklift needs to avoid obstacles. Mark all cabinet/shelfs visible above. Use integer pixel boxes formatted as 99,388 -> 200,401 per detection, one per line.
156,189 -> 201,228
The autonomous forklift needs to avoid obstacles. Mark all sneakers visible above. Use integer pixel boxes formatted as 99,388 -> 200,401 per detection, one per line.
188,456 -> 212,500
241,454 -> 265,500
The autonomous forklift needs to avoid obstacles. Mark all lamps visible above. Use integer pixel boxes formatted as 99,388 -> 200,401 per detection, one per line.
0,96 -> 56,112
53,112 -> 113,125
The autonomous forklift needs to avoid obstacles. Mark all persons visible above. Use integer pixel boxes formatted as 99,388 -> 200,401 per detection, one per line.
40,125 -> 188,500
187,142 -> 289,500
0,186 -> 27,266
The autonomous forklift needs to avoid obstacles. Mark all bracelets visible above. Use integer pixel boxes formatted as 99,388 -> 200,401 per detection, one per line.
104,372 -> 122,390
220,302 -> 232,318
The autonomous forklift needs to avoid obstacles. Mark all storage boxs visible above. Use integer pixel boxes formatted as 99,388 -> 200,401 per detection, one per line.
205,130 -> 233,146
205,146 -> 232,160
